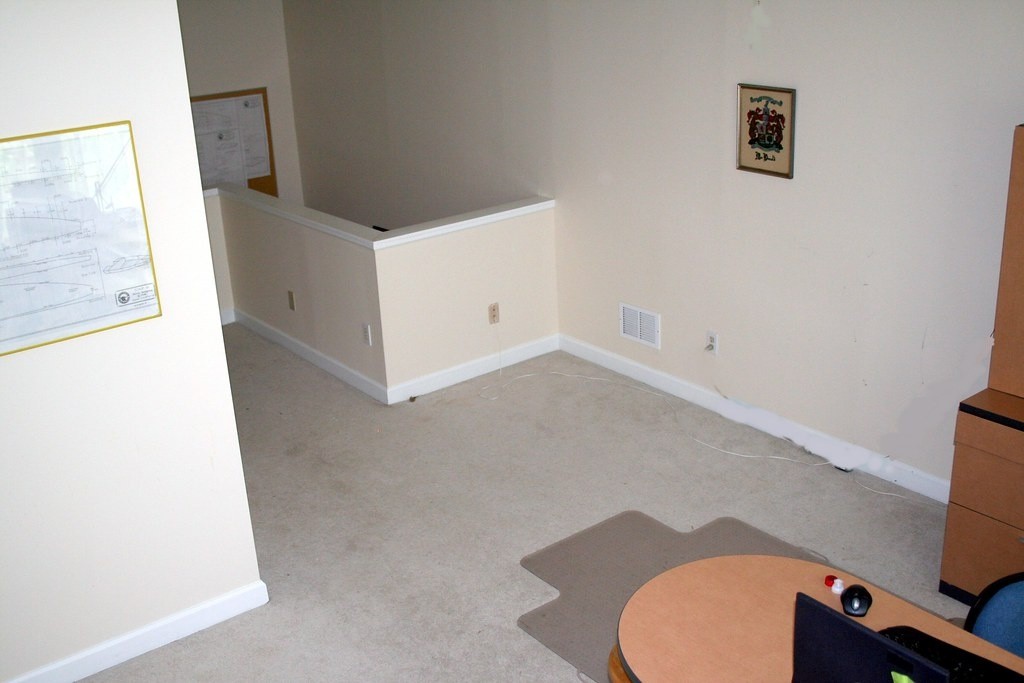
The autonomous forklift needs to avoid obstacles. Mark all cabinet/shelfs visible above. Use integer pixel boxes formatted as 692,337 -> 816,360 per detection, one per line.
938,124 -> 1024,609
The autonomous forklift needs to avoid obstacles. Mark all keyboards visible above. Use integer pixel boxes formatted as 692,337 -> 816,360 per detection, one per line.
877,625 -> 1024,683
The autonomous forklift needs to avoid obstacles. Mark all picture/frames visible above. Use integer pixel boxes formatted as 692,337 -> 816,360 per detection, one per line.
735,83 -> 796,180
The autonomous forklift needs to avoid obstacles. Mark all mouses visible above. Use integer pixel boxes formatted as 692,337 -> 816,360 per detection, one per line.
842,584 -> 873,617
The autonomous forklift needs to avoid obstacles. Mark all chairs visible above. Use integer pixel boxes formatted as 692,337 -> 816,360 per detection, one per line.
963,572 -> 1024,660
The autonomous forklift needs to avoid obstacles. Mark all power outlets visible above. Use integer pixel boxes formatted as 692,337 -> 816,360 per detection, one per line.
706,330 -> 718,353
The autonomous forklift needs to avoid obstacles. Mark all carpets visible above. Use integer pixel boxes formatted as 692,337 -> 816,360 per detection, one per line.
518,510 -> 828,683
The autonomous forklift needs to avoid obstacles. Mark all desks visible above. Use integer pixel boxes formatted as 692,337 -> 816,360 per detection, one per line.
616,556 -> 1023,683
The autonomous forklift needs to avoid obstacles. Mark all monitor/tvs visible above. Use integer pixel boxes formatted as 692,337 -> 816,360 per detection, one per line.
792,592 -> 949,683
